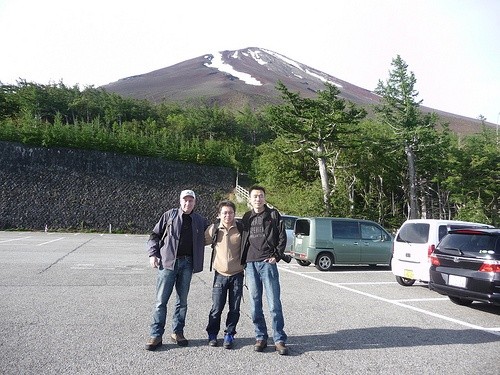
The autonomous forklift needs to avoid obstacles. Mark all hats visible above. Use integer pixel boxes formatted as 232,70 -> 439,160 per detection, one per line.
181,190 -> 195,199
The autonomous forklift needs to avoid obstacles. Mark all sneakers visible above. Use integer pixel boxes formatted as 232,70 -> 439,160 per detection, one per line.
171,332 -> 188,346
255,339 -> 267,351
275,342 -> 288,355
145,336 -> 162,350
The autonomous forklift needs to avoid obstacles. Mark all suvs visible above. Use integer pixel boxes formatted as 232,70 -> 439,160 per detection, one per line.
429,225 -> 500,306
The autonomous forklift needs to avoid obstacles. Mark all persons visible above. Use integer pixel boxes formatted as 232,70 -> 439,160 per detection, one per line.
236,184 -> 287,354
205,201 -> 244,347
144,192 -> 212,350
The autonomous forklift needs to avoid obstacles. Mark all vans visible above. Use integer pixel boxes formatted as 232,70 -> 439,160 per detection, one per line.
289,216 -> 395,272
217,214 -> 300,258
391,219 -> 496,286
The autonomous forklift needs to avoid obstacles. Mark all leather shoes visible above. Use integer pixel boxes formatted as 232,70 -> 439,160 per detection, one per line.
223,333 -> 233,348
209,334 -> 218,345
